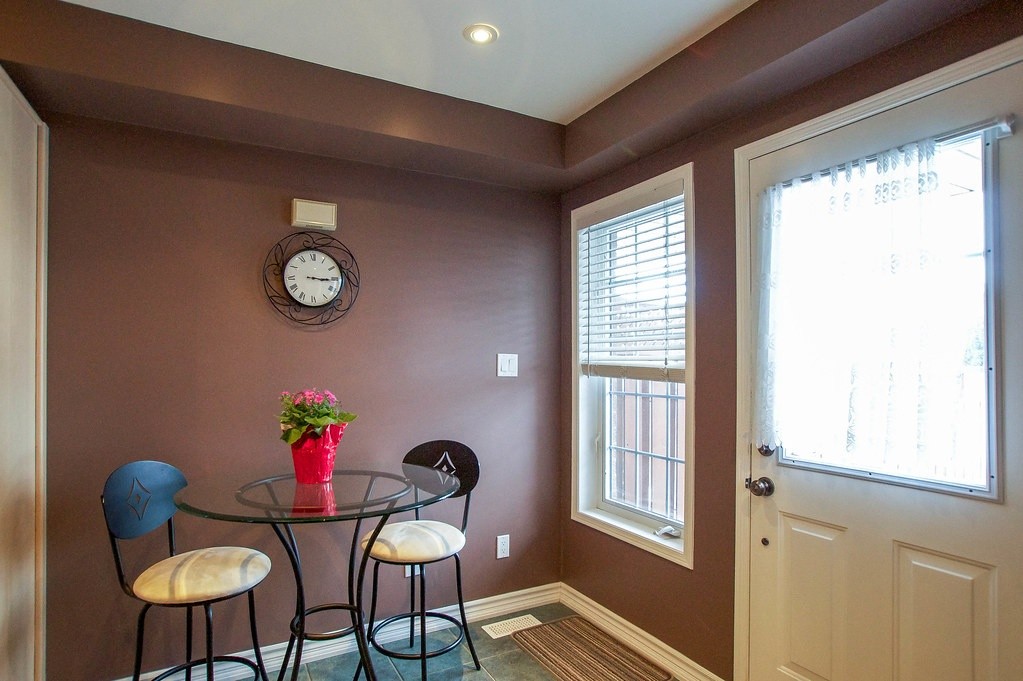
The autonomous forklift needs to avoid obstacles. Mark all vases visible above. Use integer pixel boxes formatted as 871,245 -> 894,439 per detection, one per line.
290,421 -> 348,484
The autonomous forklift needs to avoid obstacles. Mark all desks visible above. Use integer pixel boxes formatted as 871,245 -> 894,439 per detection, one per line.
175,459 -> 462,681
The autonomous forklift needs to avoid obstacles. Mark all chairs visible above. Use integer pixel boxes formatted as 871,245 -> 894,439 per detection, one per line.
101,461 -> 269,681
353,439 -> 481,681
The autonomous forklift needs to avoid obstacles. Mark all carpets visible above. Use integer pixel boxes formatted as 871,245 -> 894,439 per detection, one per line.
511,614 -> 674,681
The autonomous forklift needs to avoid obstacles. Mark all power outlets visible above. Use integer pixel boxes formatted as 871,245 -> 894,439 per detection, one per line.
497,534 -> 510,559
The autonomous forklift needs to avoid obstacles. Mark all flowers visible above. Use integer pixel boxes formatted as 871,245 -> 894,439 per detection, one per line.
276,386 -> 358,443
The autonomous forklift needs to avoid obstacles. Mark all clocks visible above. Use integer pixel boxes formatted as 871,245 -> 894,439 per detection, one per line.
284,247 -> 343,310
